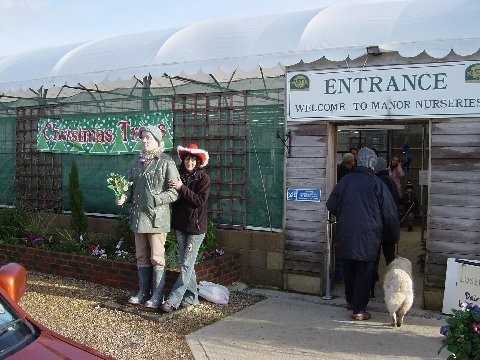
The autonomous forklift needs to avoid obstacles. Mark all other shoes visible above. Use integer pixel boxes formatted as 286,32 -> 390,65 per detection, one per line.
180,301 -> 190,307
408,224 -> 413,232
351,312 -> 371,321
161,301 -> 173,312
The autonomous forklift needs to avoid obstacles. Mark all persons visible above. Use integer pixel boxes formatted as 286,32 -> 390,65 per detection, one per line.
326,147 -> 400,321
162,144 -> 210,312
116,123 -> 181,309
337,148 -> 418,266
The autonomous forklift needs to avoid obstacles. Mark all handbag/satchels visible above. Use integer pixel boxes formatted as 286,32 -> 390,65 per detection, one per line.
197,281 -> 230,304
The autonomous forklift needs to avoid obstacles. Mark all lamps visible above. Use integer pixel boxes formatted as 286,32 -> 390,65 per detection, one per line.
366,45 -> 382,56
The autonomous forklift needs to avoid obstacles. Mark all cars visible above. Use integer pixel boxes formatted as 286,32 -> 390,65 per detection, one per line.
0,261 -> 118,360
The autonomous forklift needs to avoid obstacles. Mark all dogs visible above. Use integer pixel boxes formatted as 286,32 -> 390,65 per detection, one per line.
382,256 -> 414,328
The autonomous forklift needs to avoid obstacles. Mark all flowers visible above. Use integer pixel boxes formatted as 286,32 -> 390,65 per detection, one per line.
437,291 -> 480,360
106,172 -> 134,209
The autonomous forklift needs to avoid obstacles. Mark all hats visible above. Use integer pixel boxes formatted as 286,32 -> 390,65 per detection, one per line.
406,181 -> 413,189
176,143 -> 209,168
